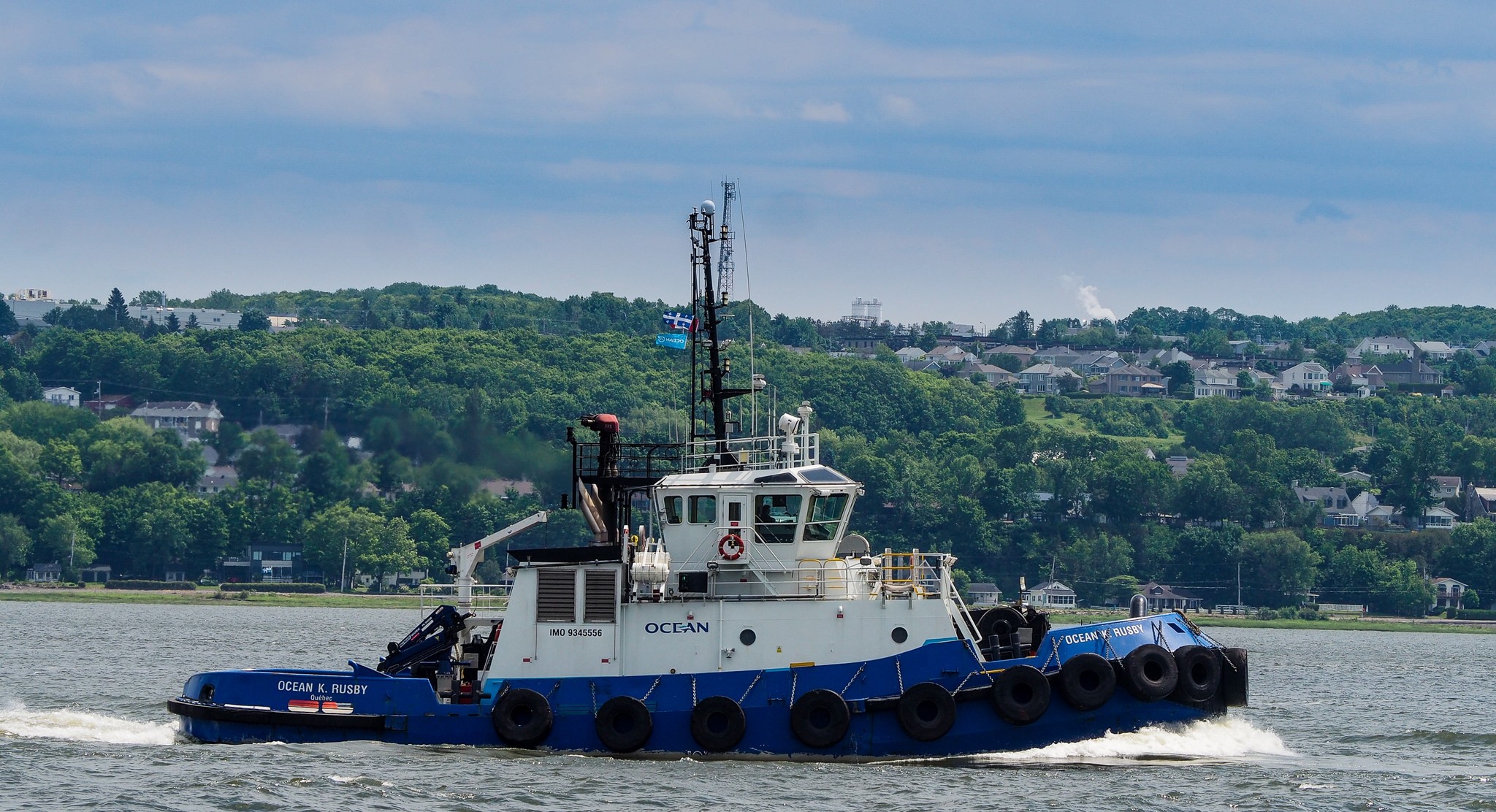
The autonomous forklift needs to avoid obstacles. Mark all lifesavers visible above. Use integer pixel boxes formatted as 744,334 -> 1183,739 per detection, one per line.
1123,644 -> 1178,699
690,696 -> 745,753
1174,645 -> 1221,702
1222,648 -> 1249,707
992,665 -> 1050,723
718,534 -> 745,561
898,683 -> 956,742
791,689 -> 850,750
1061,653 -> 1116,710
492,688 -> 552,749
595,696 -> 653,754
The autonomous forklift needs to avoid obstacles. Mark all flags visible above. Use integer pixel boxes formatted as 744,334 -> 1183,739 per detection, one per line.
662,311 -> 694,329
670,319 -> 699,333
656,334 -> 687,349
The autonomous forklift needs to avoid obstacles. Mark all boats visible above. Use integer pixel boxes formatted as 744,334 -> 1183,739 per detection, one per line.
164,176 -> 1251,758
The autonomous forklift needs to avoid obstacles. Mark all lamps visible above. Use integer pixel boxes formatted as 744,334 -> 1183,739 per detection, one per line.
778,413 -> 800,452
860,556 -> 872,567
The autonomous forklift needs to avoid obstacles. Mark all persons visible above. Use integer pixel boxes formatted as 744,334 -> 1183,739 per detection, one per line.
761,505 -> 775,523
